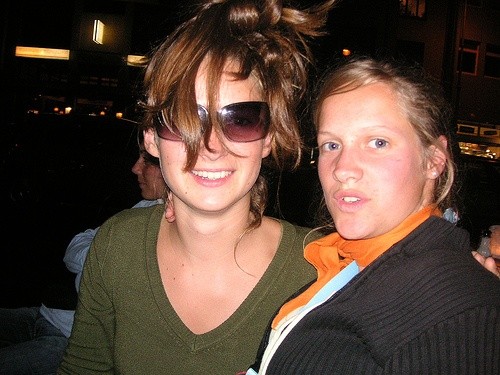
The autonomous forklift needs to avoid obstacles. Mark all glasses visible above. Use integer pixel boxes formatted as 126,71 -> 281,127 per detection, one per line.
153,101 -> 272,143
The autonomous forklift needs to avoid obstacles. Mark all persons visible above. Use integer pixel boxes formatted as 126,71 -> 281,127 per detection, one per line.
0,124 -> 170,375
162,46 -> 500,375
52,0 -> 500,375
